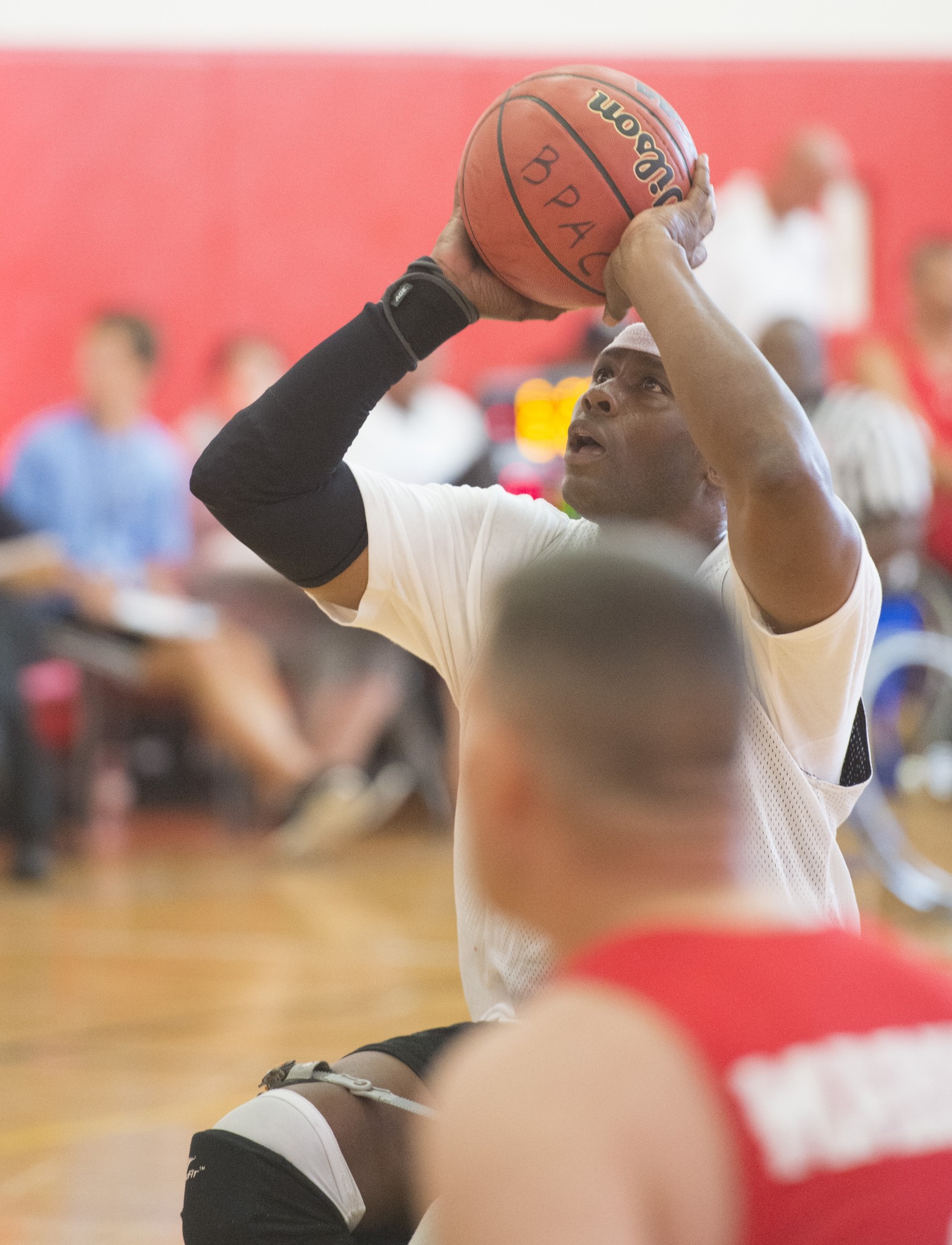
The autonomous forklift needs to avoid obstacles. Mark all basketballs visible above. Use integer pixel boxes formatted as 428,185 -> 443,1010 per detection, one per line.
457,64 -> 699,308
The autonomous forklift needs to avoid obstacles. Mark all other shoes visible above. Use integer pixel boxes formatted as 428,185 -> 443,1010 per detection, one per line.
271,763 -> 420,858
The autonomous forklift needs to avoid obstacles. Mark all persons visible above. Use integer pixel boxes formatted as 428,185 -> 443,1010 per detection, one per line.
412,550 -> 952,1245
0,237 -> 952,881
179,164 -> 881,1245
